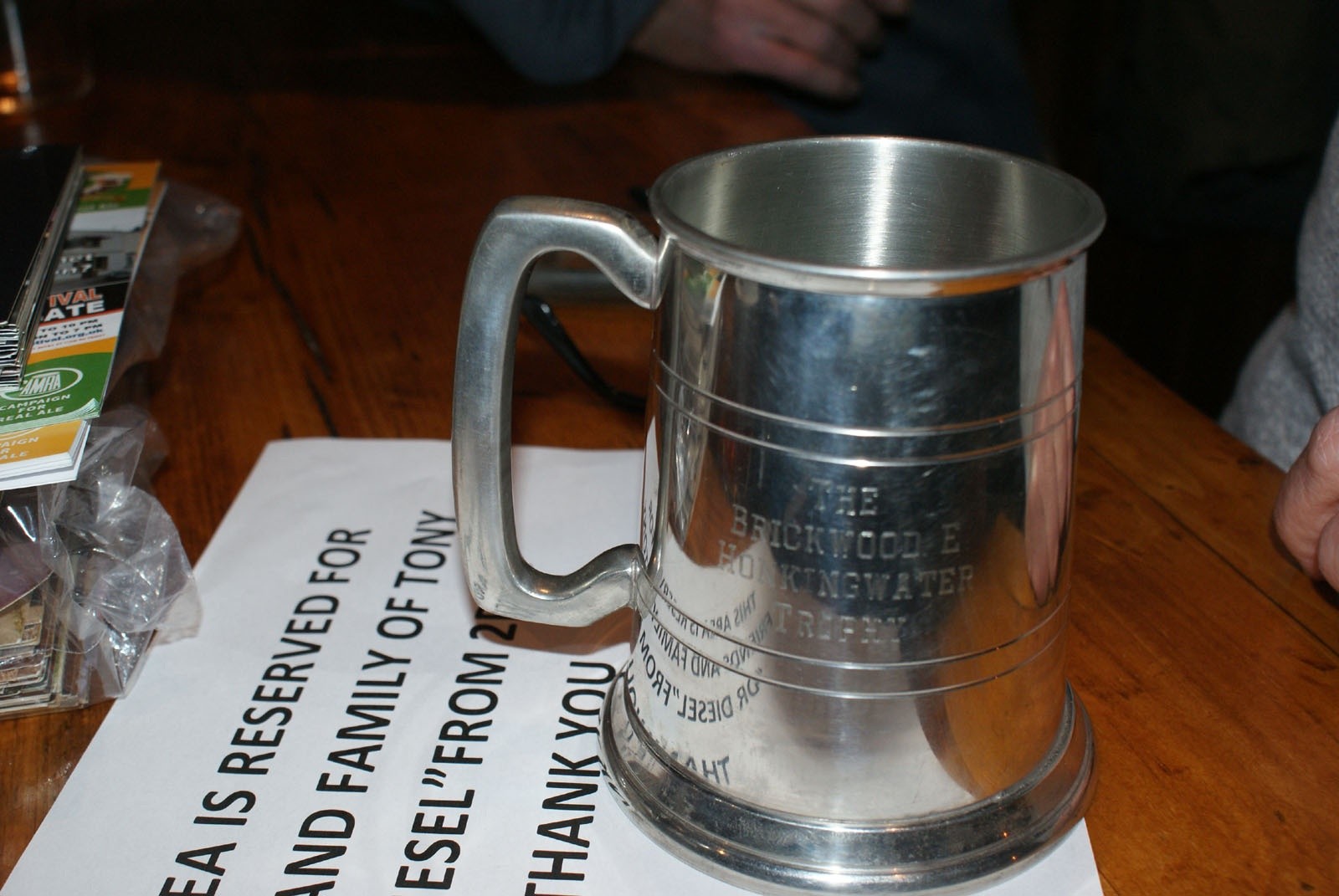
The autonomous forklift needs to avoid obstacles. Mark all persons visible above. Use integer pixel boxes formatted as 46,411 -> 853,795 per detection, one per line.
1218,120 -> 1339,594
460,0 -> 1043,159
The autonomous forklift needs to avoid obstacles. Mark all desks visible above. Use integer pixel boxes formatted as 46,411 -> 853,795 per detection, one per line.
0,0 -> 1339,896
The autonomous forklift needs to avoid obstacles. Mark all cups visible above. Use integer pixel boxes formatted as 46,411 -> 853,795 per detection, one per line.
451,135 -> 1100,896
1,0 -> 112,179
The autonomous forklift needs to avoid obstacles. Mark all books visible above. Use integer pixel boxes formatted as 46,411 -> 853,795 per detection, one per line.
0,159 -> 168,490
